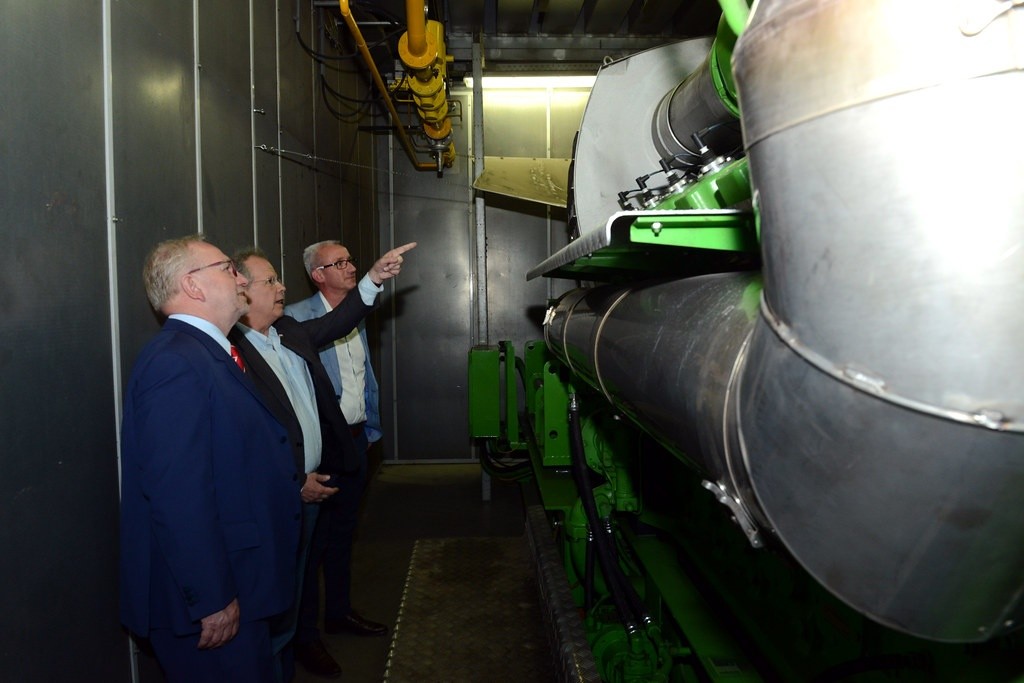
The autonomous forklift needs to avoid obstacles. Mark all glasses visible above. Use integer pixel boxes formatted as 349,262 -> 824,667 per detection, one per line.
317,257 -> 357,270
187,259 -> 238,278
253,276 -> 280,287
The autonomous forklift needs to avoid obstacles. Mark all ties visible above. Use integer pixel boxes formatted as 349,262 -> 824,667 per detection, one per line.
231,344 -> 247,374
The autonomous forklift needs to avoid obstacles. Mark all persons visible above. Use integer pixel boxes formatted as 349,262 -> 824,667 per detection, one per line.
285,241 -> 387,675
226,242 -> 418,545
119,235 -> 300,683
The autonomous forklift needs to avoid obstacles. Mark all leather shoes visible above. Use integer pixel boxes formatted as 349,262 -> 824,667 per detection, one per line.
295,638 -> 343,680
322,612 -> 388,635
